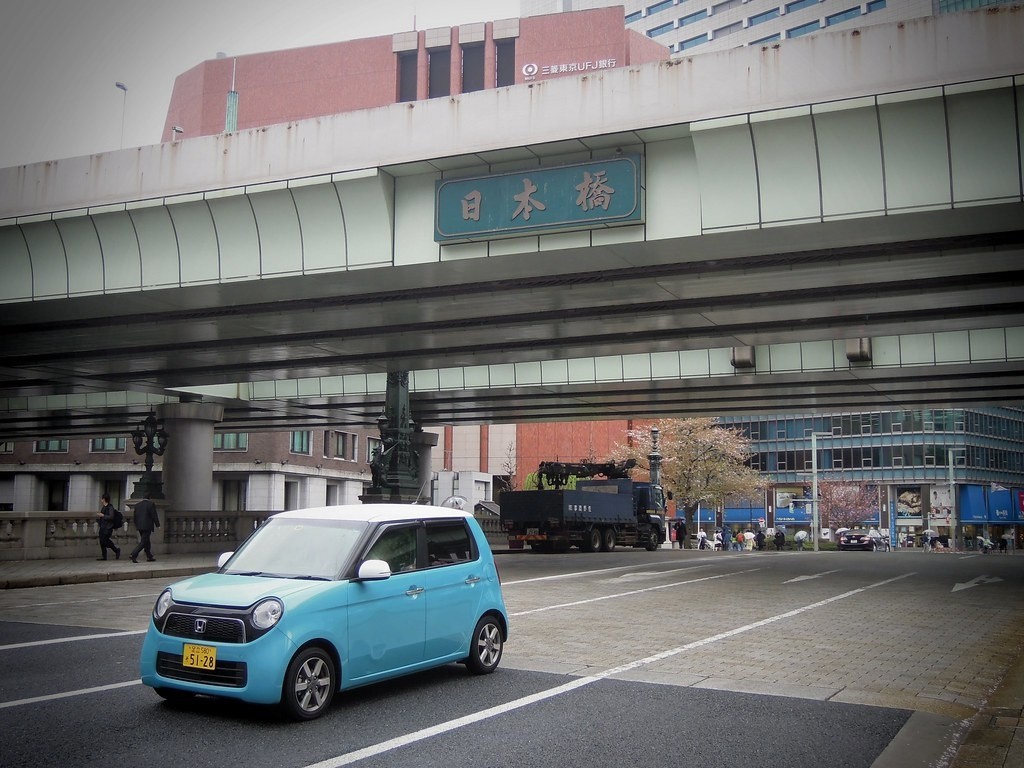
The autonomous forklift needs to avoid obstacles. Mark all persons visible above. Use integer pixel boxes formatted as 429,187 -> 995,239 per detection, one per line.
96,494 -> 120,560
839,532 -> 843,550
129,491 -> 160,563
999,537 -> 1007,553
923,532 -> 941,552
978,539 -> 993,554
775,529 -> 785,551
411,500 -> 430,505
714,530 -> 766,552
674,520 -> 687,549
474,513 -> 478,520
697,527 -> 706,550
932,489 -> 951,514
797,536 -> 803,551
454,501 -> 463,510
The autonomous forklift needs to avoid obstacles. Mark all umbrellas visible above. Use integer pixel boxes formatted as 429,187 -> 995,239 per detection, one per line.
745,533 -> 755,539
669,517 -> 685,521
924,530 -> 934,532
713,526 -> 731,530
441,495 -> 468,508
794,531 -> 807,541
977,537 -> 984,541
835,528 -> 849,534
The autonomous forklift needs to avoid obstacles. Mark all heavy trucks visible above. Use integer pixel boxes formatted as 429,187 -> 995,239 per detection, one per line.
500,459 -> 673,553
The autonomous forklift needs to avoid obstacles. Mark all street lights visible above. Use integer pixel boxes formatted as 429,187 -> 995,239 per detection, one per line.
132,403 -> 169,501
811,431 -> 833,552
171,125 -> 184,141
115,81 -> 128,149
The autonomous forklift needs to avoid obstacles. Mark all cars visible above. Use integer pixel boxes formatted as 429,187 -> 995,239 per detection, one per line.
839,528 -> 891,554
141,502 -> 511,720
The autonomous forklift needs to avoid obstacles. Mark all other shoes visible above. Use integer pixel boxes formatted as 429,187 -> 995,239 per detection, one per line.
147,557 -> 156,561
96,558 -> 107,561
116,548 -> 121,559
129,555 -> 138,563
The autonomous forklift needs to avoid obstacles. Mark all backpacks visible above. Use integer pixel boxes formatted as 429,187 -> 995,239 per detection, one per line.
113,509 -> 123,529
